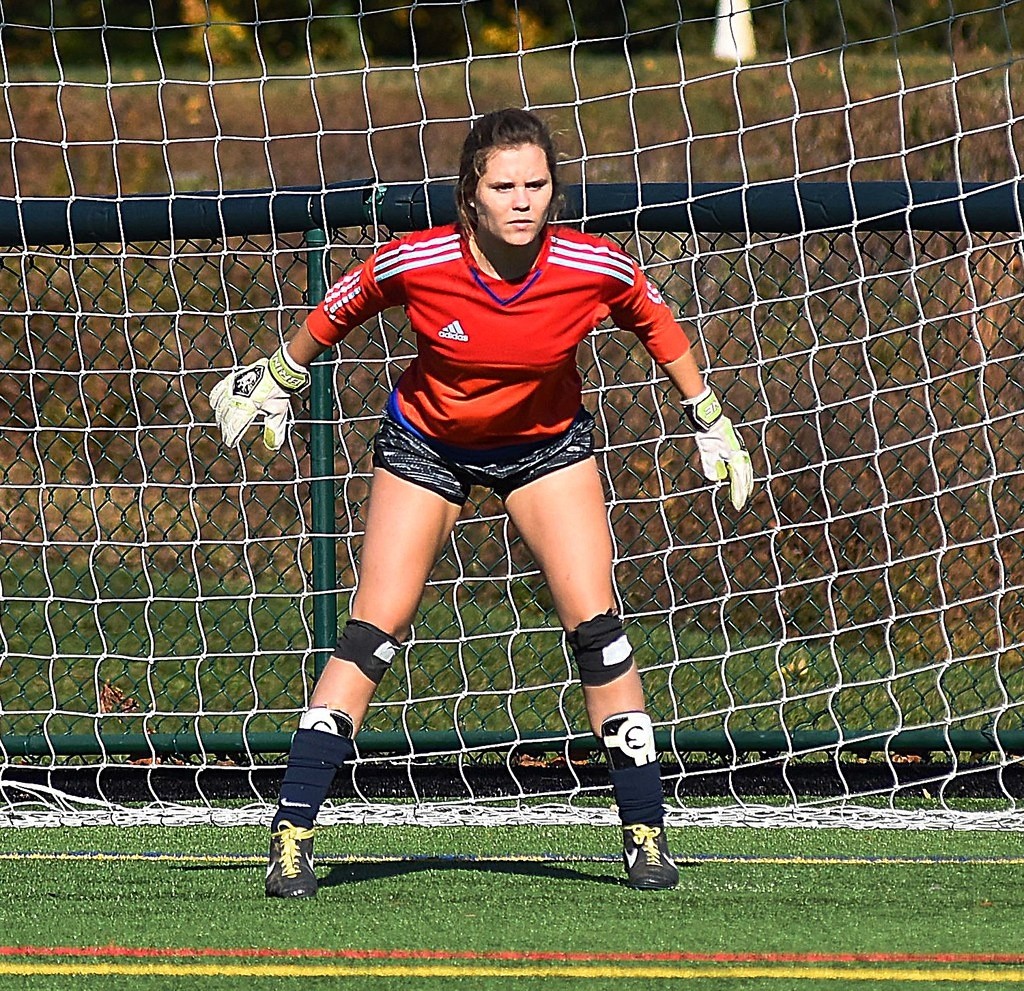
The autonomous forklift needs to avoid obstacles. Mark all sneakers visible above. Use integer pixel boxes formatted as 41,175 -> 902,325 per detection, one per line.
620,817 -> 681,890
263,820 -> 321,901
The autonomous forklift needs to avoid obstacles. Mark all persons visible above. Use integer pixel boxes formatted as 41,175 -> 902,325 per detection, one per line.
208,108 -> 754,899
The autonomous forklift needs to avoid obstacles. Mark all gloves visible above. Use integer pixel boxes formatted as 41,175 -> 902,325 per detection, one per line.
679,389 -> 755,513
207,338 -> 312,453
